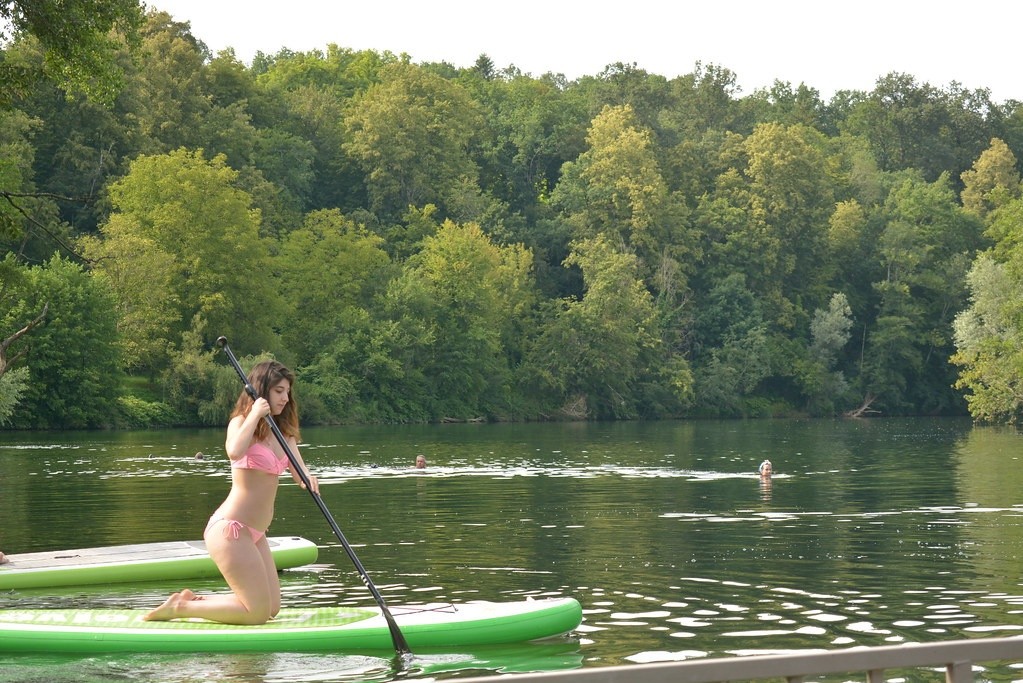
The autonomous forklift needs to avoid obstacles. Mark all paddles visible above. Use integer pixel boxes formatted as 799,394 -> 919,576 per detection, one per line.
217,336 -> 411,657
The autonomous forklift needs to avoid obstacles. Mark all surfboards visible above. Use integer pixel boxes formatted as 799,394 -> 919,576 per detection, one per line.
1,596 -> 584,654
0,535 -> 320,593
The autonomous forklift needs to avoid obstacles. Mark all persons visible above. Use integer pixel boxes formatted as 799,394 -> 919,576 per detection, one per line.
142,362 -> 320,625
415,455 -> 426,469
758,460 -> 772,475
